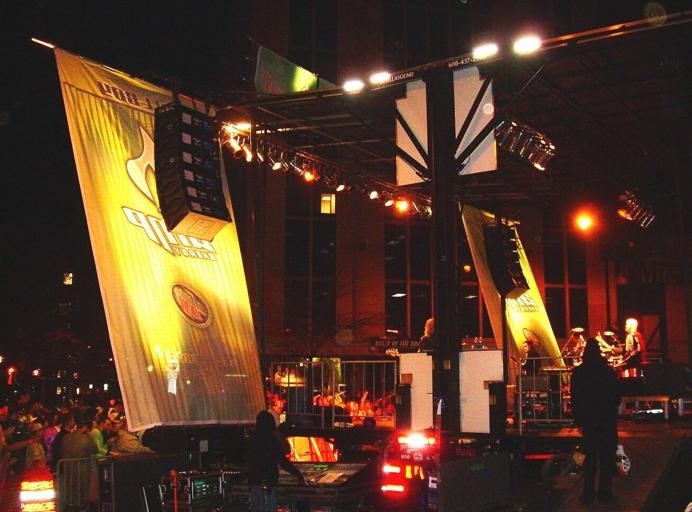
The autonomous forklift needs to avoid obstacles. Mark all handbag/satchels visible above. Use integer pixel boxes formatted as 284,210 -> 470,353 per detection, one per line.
615,445 -> 632,476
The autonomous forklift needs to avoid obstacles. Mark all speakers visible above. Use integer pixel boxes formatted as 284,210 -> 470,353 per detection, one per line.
280,386 -> 352,430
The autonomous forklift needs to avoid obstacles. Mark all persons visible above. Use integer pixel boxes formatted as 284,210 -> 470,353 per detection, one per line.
517,318 -> 641,380
0,383 -> 159,481
416,318 -> 435,352
245,381 -> 399,511
569,336 -> 623,506
